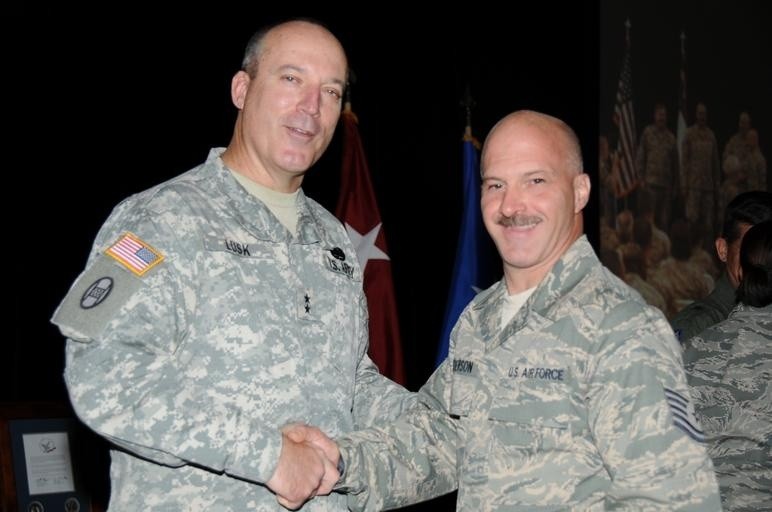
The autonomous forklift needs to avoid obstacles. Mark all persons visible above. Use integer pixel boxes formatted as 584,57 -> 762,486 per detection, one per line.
670,190 -> 771,344
50,20 -> 418,511
283,109 -> 723,512
598,106 -> 767,319
683,223 -> 771,512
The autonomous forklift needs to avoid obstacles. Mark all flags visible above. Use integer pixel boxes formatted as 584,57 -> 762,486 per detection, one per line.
434,135 -> 478,370
614,38 -> 640,198
674,48 -> 688,167
332,112 -> 405,384
